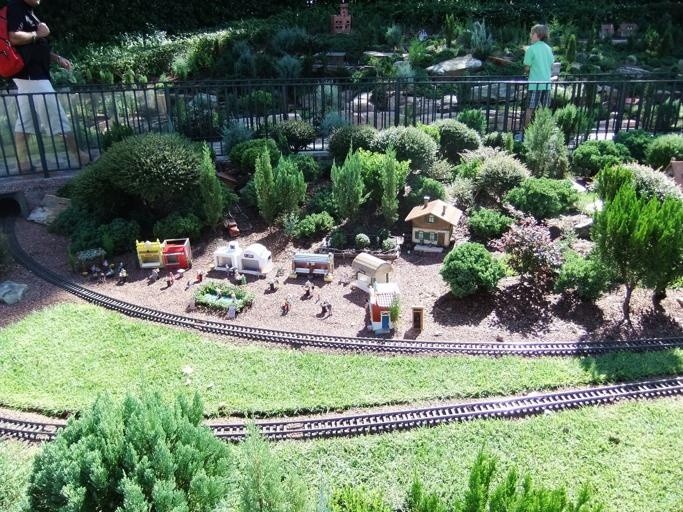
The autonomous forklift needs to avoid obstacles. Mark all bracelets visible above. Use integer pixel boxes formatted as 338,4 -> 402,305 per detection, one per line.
32,31 -> 37,43
55,56 -> 61,65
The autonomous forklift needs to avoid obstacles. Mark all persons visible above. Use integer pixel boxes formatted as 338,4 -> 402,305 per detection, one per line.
90,258 -> 205,287
222,263 -> 332,319
512,23 -> 554,142
6,0 -> 101,175
